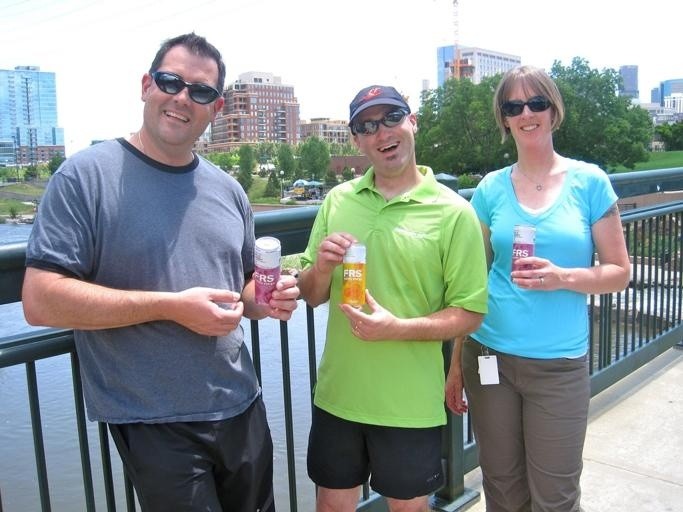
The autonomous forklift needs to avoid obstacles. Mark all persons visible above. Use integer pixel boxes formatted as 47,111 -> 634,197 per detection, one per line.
443,64 -> 633,512
21,30 -> 301,510
286,185 -> 324,199
295,84 -> 489,512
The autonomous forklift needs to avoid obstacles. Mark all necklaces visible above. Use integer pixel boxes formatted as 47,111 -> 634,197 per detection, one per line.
137,131 -> 194,164
514,158 -> 559,191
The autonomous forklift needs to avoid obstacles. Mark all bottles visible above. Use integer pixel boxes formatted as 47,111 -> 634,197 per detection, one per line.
341,244 -> 366,307
254,236 -> 282,305
511,224 -> 536,282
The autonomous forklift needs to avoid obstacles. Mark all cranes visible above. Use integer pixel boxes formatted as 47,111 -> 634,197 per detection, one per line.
444,0 -> 464,82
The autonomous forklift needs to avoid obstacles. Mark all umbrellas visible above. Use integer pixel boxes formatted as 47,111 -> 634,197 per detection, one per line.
307,180 -> 323,186
293,179 -> 309,188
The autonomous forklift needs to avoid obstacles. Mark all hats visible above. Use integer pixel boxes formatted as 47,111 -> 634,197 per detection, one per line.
347,86 -> 411,127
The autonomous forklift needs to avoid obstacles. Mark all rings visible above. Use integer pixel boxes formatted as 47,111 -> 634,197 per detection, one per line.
540,277 -> 544,284
358,320 -> 363,328
354,326 -> 358,329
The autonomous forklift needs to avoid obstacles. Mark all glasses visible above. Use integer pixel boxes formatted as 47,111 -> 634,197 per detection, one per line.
352,109 -> 408,134
502,95 -> 551,117
151,71 -> 222,104
